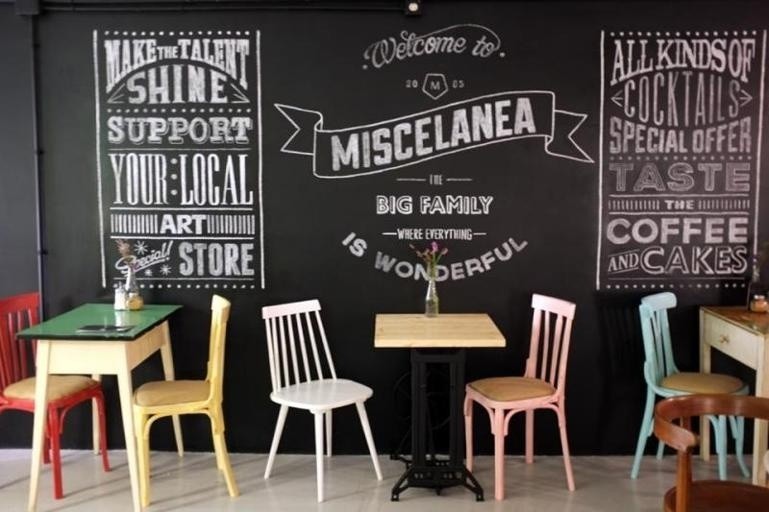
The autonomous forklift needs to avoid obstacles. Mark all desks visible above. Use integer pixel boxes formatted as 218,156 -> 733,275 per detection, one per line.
12,301 -> 189,511
689,299 -> 769,489
367,312 -> 506,504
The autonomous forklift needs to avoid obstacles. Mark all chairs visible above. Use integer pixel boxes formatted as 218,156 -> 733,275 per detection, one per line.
1,289 -> 114,501
461,292 -> 577,502
262,298 -> 384,505
645,389 -> 769,511
128,294 -> 239,509
629,290 -> 755,483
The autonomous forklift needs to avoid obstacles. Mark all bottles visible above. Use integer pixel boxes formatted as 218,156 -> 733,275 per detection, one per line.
114,312 -> 129,332
127,291 -> 145,311
749,294 -> 769,313
112,281 -> 128,310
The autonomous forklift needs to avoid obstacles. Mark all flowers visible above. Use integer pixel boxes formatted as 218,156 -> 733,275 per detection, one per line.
415,240 -> 449,315
124,258 -> 140,271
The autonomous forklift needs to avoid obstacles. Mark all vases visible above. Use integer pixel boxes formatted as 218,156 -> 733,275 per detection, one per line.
125,267 -> 139,296
421,274 -> 441,317
